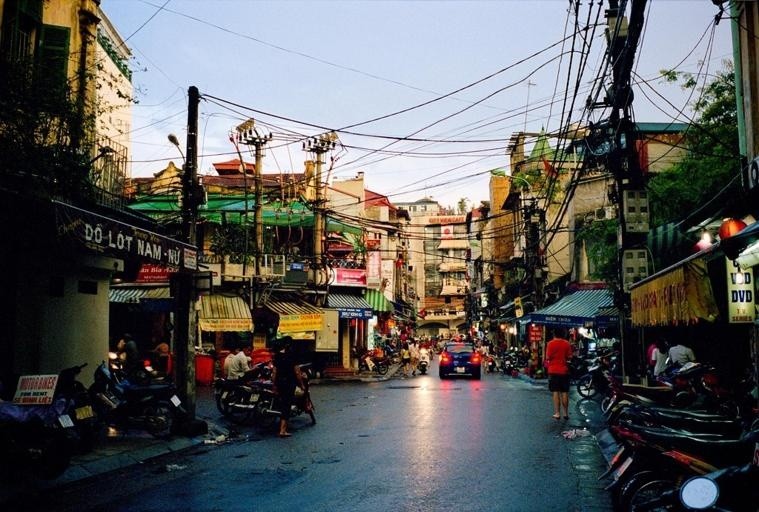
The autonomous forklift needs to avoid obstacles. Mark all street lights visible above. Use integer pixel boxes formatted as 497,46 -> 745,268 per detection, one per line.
485,166 -> 546,371
163,128 -> 198,433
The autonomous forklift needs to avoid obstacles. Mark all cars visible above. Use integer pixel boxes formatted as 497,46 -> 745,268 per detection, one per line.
438,337 -> 450,351
482,330 -> 492,345
437,341 -> 481,381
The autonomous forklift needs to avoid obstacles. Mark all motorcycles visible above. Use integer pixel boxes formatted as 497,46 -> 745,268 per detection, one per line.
566,342 -> 759,512
485,344 -> 529,375
361,335 -> 437,378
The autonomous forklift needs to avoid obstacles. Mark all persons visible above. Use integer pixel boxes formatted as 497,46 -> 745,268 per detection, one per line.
400,337 -> 419,376
224,344 -> 259,380
116,334 -> 138,366
648,338 -> 696,376
272,336 -> 305,436
546,327 -> 573,418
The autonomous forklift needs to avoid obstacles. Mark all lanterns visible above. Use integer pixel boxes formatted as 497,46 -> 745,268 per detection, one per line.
694,241 -> 712,252
719,219 -> 747,240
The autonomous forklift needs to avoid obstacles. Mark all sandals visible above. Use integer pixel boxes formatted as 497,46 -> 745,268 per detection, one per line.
280,432 -> 292,438
